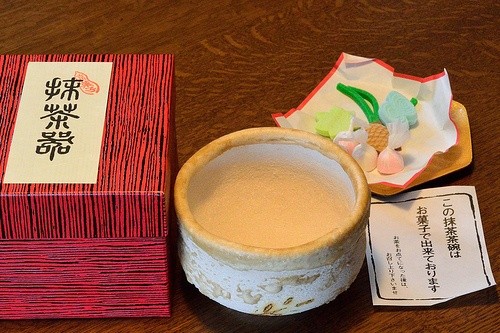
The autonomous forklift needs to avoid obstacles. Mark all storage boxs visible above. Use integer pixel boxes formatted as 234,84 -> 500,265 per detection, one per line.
0,54 -> 178,321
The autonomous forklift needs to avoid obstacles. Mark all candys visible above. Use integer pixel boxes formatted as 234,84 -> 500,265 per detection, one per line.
315,91 -> 418,175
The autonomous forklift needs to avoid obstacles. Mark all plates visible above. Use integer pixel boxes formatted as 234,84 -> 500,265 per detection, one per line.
367,99 -> 473,197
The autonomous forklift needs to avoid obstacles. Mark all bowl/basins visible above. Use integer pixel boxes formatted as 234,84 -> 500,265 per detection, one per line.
173,125 -> 370,316
